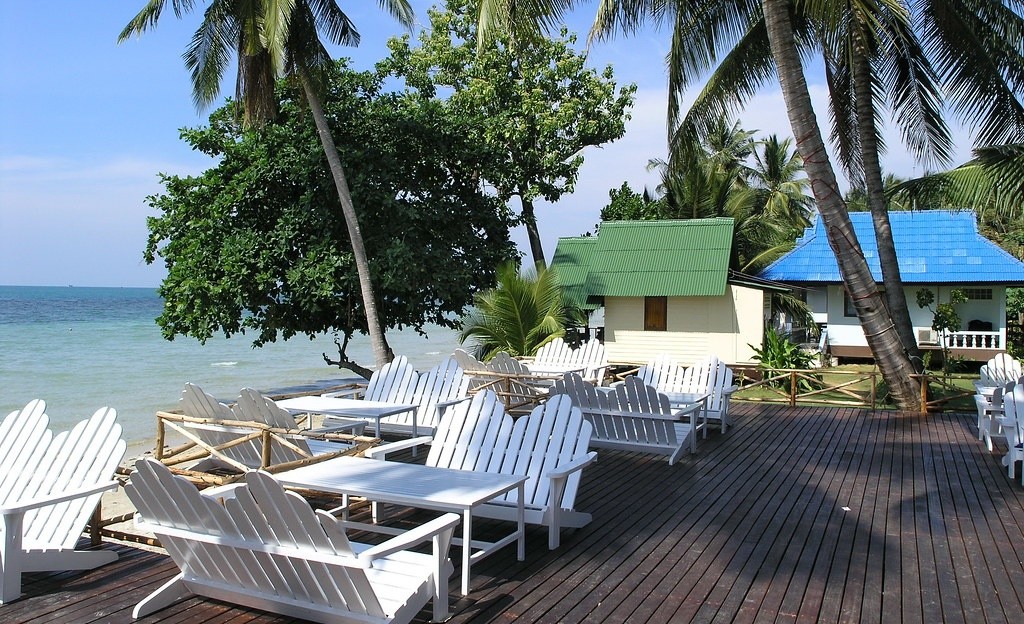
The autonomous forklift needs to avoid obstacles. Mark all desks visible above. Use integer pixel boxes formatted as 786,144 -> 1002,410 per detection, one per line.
274,396 -> 421,461
484,361 -> 588,388
972,380 -> 1007,434
578,386 -> 711,453
273,455 -> 530,595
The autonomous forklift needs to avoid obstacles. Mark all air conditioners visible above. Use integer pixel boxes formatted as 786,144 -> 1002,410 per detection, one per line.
918,329 -> 938,344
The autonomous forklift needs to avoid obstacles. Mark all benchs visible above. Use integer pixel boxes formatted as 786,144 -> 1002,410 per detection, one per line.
0,338 -> 741,624
980,352 -> 1024,486
